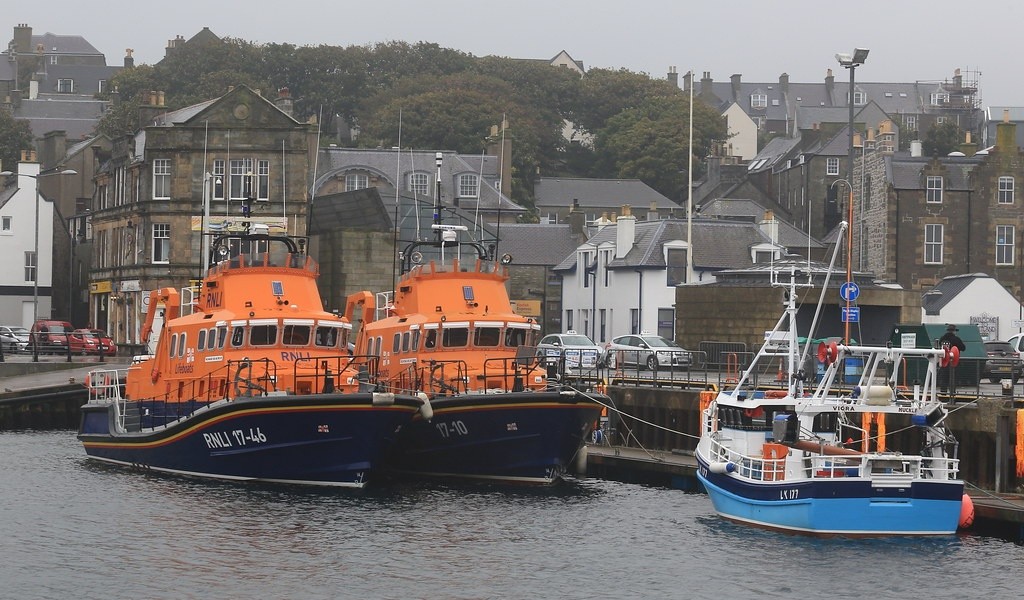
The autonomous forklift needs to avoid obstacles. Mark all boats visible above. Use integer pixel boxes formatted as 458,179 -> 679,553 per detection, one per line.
694,201 -> 964,534
345,107 -> 610,488
77,103 -> 425,495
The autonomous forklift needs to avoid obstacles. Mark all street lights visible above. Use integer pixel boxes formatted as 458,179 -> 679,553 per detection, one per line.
835,48 -> 870,345
204,171 -> 221,277
686,69 -> 695,285
860,140 -> 876,271
0,169 -> 77,362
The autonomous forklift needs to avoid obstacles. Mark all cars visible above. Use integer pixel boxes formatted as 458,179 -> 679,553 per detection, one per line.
68,329 -> 116,357
536,330 -> 605,369
0,326 -> 31,354
1006,333 -> 1024,361
603,330 -> 694,371
982,341 -> 1024,383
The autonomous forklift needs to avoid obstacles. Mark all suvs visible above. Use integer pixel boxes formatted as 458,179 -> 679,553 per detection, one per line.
29,320 -> 75,355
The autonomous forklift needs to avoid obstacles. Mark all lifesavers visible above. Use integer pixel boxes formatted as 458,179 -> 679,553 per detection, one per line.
85,368 -> 110,395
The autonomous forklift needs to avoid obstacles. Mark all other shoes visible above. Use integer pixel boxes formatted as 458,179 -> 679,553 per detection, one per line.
952,392 -> 956,395
941,391 -> 947,395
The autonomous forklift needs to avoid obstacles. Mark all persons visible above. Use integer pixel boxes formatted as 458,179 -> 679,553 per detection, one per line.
605,342 -> 611,350
940,324 -> 966,396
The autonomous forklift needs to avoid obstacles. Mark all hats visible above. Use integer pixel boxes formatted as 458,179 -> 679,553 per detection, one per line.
946,324 -> 959,332
